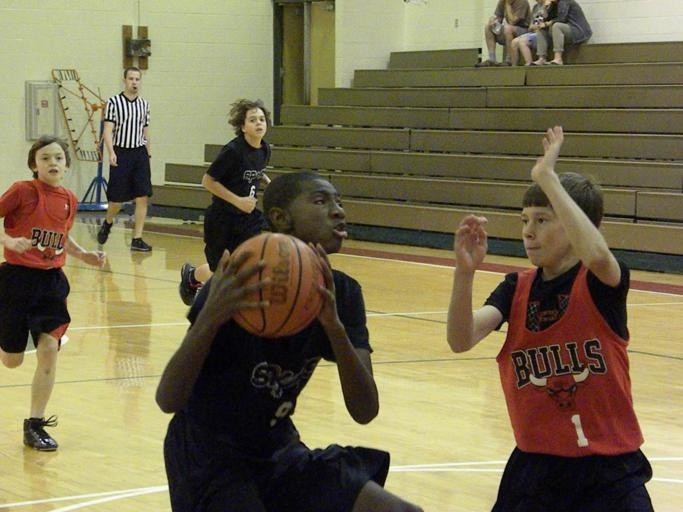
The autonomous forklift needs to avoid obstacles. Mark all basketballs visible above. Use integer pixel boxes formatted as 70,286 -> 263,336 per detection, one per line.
224,233 -> 326,340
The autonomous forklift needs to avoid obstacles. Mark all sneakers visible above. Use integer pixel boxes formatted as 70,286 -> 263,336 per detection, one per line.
98,219 -> 113,245
475,59 -> 563,67
22,417 -> 59,449
181,264 -> 198,305
132,237 -> 153,251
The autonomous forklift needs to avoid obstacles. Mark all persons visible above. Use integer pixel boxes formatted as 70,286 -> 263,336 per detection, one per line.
176,97 -> 272,305
530,0 -> 592,65
510,0 -> 550,65
95,66 -> 151,251
0,135 -> 106,451
444,124 -> 655,512
474,0 -> 530,66
155,170 -> 423,512
